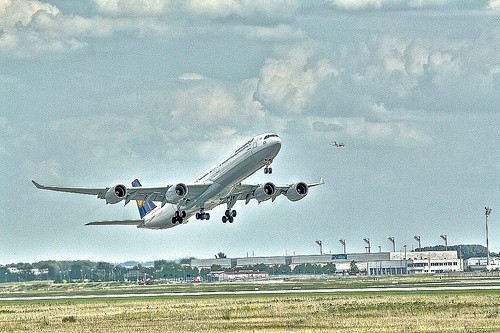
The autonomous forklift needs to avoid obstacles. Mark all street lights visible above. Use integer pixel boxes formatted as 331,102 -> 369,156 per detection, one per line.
485,206 -> 492,265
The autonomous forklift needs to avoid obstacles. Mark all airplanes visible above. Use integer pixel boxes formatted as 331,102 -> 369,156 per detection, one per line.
31,132 -> 325,231
